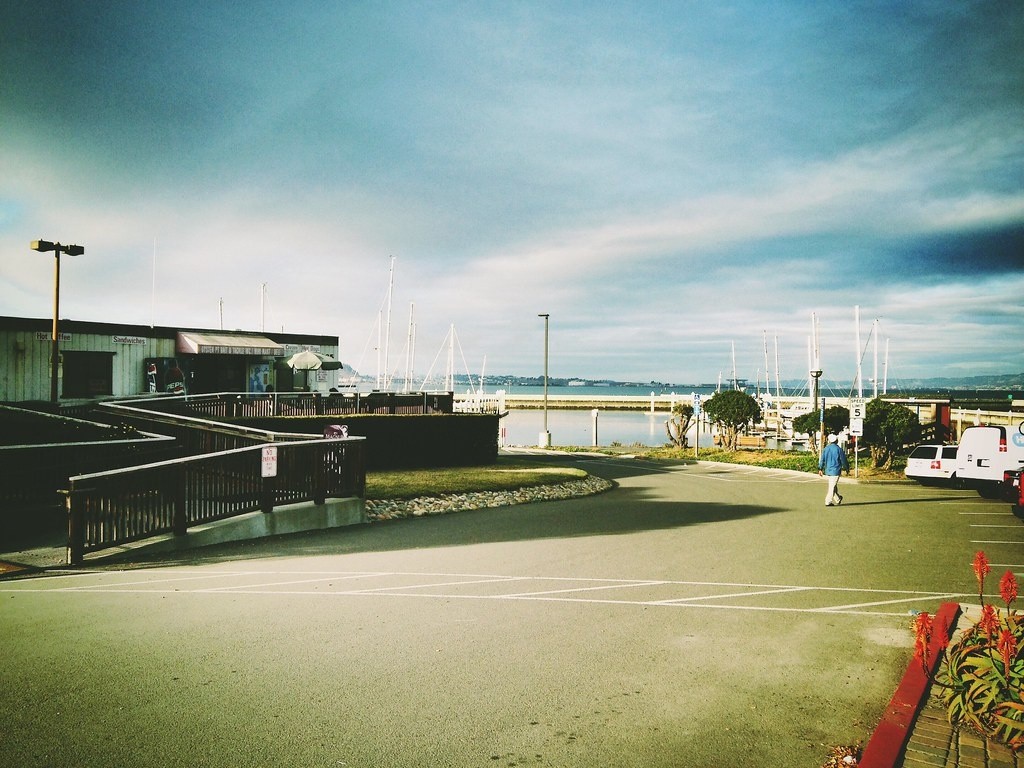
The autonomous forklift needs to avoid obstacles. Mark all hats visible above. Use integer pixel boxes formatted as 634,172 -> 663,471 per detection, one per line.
827,434 -> 838,443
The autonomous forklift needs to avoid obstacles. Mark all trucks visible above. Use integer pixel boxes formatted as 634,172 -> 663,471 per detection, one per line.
956,425 -> 1024,499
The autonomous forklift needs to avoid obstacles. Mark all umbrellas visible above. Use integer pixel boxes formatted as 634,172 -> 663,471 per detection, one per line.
273,350 -> 344,384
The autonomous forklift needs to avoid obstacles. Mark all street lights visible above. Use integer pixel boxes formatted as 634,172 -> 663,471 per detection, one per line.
811,370 -> 822,449
538,314 -> 551,447
31,239 -> 85,403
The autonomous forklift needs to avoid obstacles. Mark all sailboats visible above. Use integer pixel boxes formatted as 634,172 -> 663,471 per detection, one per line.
417,325 -> 482,415
780,313 -> 855,418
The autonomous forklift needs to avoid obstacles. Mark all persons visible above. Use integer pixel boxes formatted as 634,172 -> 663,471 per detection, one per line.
326,388 -> 345,408
819,434 -> 851,507
298,386 -> 313,409
261,385 -> 278,398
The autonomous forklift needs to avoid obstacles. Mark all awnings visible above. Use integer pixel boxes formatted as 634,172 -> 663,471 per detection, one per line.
174,331 -> 285,356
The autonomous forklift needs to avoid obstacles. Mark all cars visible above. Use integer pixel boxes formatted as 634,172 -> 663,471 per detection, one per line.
904,441 -> 973,489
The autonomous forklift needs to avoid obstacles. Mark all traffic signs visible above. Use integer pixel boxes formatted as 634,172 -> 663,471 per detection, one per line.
693,394 -> 700,415
849,418 -> 863,437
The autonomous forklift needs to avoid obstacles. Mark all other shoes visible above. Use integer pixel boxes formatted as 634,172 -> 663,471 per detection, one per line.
826,503 -> 834,506
837,495 -> 843,505
